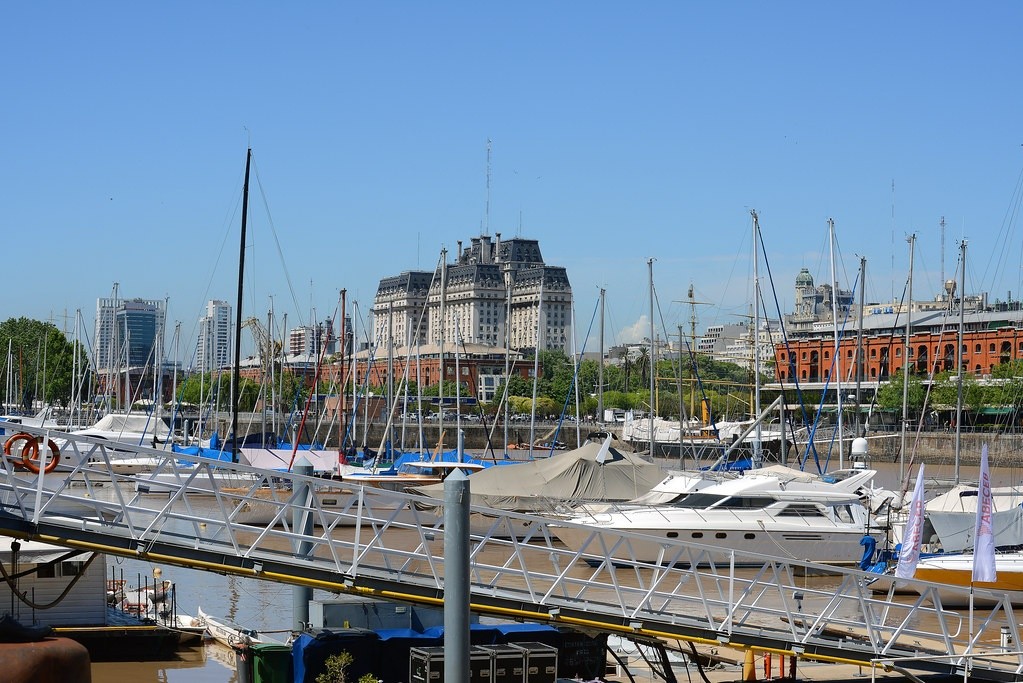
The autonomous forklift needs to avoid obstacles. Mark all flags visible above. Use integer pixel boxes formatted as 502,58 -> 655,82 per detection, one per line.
895,461 -> 924,588
971,443 -> 997,583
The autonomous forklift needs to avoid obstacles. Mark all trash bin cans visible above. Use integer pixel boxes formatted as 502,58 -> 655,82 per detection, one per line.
250,643 -> 292,683
230,642 -> 261,683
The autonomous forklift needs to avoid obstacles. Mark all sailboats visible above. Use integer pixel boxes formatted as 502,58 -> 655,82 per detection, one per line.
0,140 -> 1022,683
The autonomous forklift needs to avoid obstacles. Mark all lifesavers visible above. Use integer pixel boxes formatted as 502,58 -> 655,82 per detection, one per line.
22,437 -> 60,474
3,433 -> 39,468
